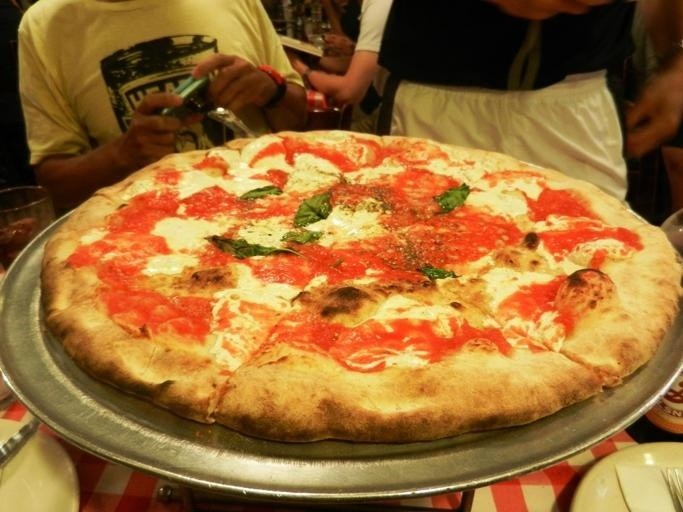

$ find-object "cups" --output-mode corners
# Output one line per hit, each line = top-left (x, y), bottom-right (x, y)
(2, 186), (54, 261)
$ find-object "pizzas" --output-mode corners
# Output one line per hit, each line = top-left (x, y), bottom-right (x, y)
(40, 128), (682, 443)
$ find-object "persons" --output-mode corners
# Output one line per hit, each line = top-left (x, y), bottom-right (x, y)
(17, 1), (681, 221)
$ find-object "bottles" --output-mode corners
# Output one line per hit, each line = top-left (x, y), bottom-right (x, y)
(642, 201), (682, 432)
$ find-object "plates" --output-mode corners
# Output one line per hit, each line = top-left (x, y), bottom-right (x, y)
(571, 441), (681, 512)
(0, 418), (83, 512)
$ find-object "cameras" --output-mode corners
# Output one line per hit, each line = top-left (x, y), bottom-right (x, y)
(158, 72), (213, 122)
